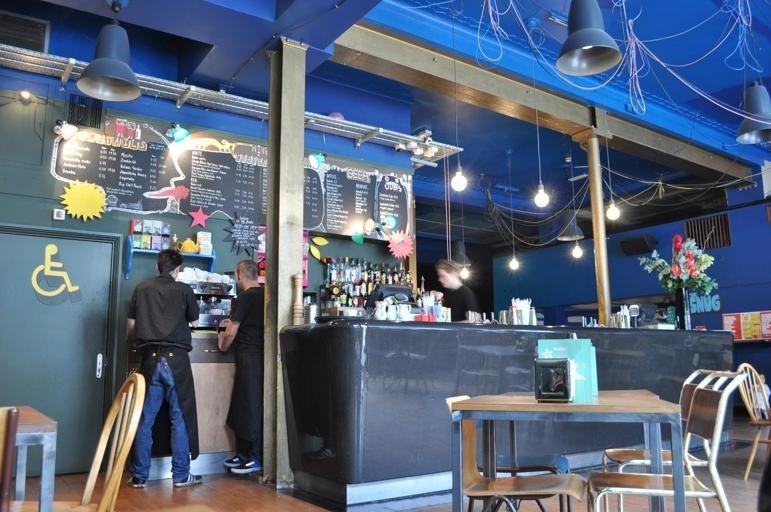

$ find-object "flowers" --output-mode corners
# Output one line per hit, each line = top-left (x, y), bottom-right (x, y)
(638, 233), (719, 296)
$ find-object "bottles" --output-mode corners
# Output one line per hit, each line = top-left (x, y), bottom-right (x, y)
(171, 234), (178, 253)
(509, 305), (519, 327)
(415, 288), (421, 307)
(619, 305), (630, 328)
(324, 256), (410, 307)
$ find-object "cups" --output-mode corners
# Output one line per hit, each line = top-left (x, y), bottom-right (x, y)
(373, 301), (388, 321)
(498, 310), (507, 325)
(397, 304), (411, 321)
(388, 304), (400, 320)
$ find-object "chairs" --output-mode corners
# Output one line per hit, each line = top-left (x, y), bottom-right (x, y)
(585, 369), (749, 511)
(0, 407), (19, 512)
(9, 373), (146, 512)
(445, 394), (590, 511)
(736, 363), (770, 483)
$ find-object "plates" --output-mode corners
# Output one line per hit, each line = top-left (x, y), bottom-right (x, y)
(313, 315), (364, 324)
(304, 312), (317, 325)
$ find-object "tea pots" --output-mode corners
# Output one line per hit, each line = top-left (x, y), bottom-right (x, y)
(178, 238), (199, 254)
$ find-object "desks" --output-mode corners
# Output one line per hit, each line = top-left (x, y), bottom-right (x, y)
(452, 387), (687, 511)
(0, 405), (58, 512)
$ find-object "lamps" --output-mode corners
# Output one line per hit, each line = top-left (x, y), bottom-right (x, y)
(170, 122), (189, 142)
(352, 216), (396, 244)
(555, 1), (622, 77)
(76, 0), (142, 103)
(556, 209), (584, 242)
(309, 133), (328, 169)
(57, 120), (78, 140)
(736, 57), (771, 145)
(453, 241), (471, 268)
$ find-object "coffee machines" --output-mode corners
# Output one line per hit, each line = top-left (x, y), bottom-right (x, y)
(187, 281), (236, 330)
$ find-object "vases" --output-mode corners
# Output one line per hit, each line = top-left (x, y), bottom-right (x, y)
(677, 288), (691, 333)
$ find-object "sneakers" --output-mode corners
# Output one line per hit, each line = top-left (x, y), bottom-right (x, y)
(224, 456), (243, 467)
(231, 460), (263, 474)
(127, 477), (146, 488)
(173, 473), (203, 486)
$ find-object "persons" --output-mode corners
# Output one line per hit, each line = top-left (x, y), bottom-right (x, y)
(754, 374), (771, 421)
(127, 249), (206, 488)
(435, 258), (477, 322)
(217, 259), (264, 476)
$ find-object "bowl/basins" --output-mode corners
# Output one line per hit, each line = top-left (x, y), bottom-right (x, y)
(175, 267), (231, 282)
